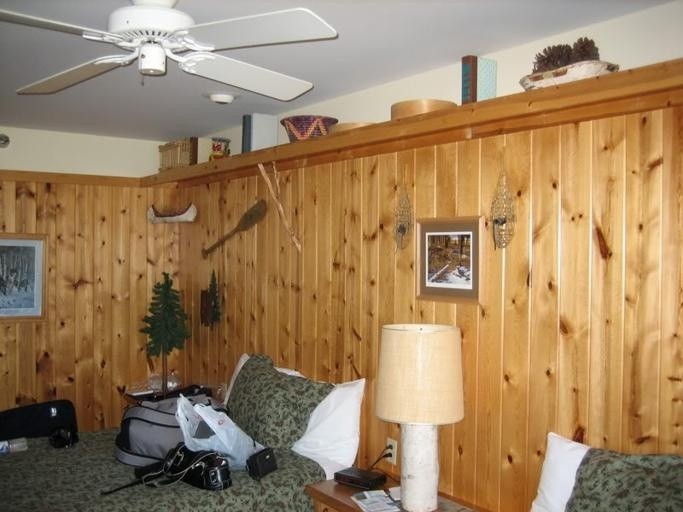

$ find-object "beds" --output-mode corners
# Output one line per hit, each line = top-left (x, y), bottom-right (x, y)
(0, 425), (340, 511)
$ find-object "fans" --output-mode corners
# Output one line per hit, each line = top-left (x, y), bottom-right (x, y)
(0, 0), (338, 102)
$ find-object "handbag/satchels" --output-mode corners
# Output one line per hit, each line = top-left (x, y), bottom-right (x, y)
(165, 440), (232, 490)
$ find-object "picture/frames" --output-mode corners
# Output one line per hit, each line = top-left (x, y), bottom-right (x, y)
(415, 217), (480, 304)
(0, 231), (48, 322)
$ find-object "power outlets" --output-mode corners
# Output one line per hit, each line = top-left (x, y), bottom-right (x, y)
(384, 437), (397, 467)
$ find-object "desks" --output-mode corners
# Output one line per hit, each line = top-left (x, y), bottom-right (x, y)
(124, 387), (211, 408)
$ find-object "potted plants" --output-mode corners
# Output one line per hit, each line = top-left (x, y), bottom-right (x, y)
(520, 36), (621, 91)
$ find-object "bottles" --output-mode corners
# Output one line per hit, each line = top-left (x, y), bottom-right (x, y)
(0, 437), (28, 454)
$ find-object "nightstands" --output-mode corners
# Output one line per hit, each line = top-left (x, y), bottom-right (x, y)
(304, 479), (490, 512)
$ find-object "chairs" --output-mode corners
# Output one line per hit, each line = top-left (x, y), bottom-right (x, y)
(1, 399), (77, 440)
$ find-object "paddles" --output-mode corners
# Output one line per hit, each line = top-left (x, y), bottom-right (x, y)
(201, 201), (266, 259)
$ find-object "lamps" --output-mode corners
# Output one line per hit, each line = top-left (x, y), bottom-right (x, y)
(375, 323), (466, 512)
(492, 171), (517, 249)
(392, 178), (412, 250)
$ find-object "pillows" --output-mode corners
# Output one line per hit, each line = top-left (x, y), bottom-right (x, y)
(222, 351), (366, 481)
(523, 432), (683, 512)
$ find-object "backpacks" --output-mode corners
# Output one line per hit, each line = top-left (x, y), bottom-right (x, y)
(115, 393), (229, 467)
